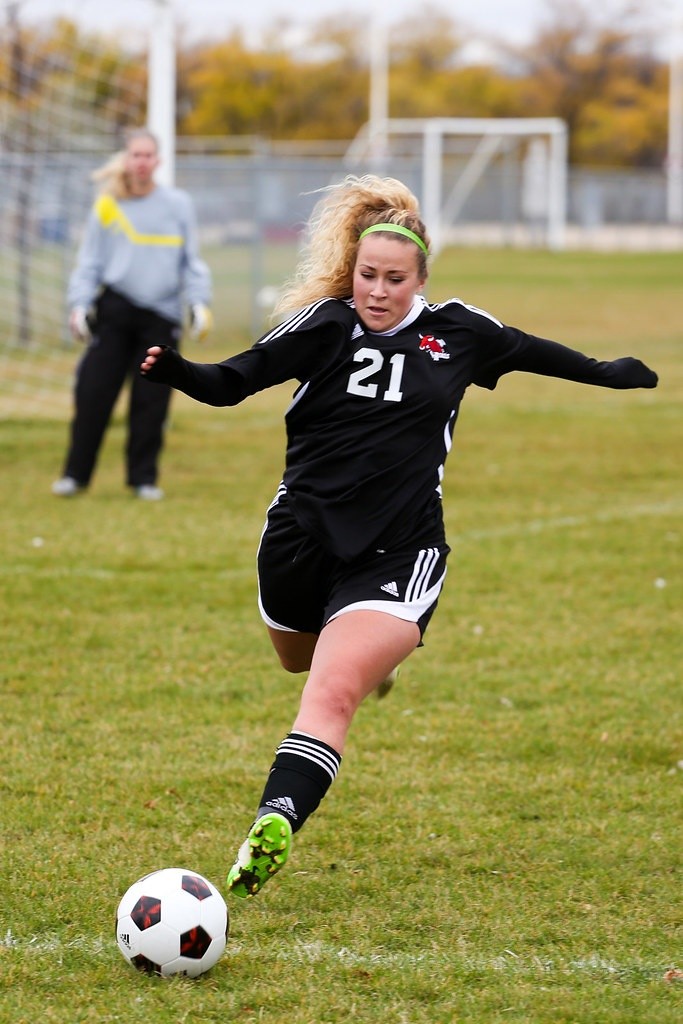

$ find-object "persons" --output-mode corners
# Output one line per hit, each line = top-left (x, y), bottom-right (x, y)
(140, 172), (659, 898)
(51, 130), (211, 499)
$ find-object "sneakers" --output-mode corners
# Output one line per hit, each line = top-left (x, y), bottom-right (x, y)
(135, 485), (163, 500)
(50, 476), (81, 496)
(226, 810), (292, 898)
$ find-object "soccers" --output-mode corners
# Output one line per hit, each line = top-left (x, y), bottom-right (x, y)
(115, 867), (231, 978)
